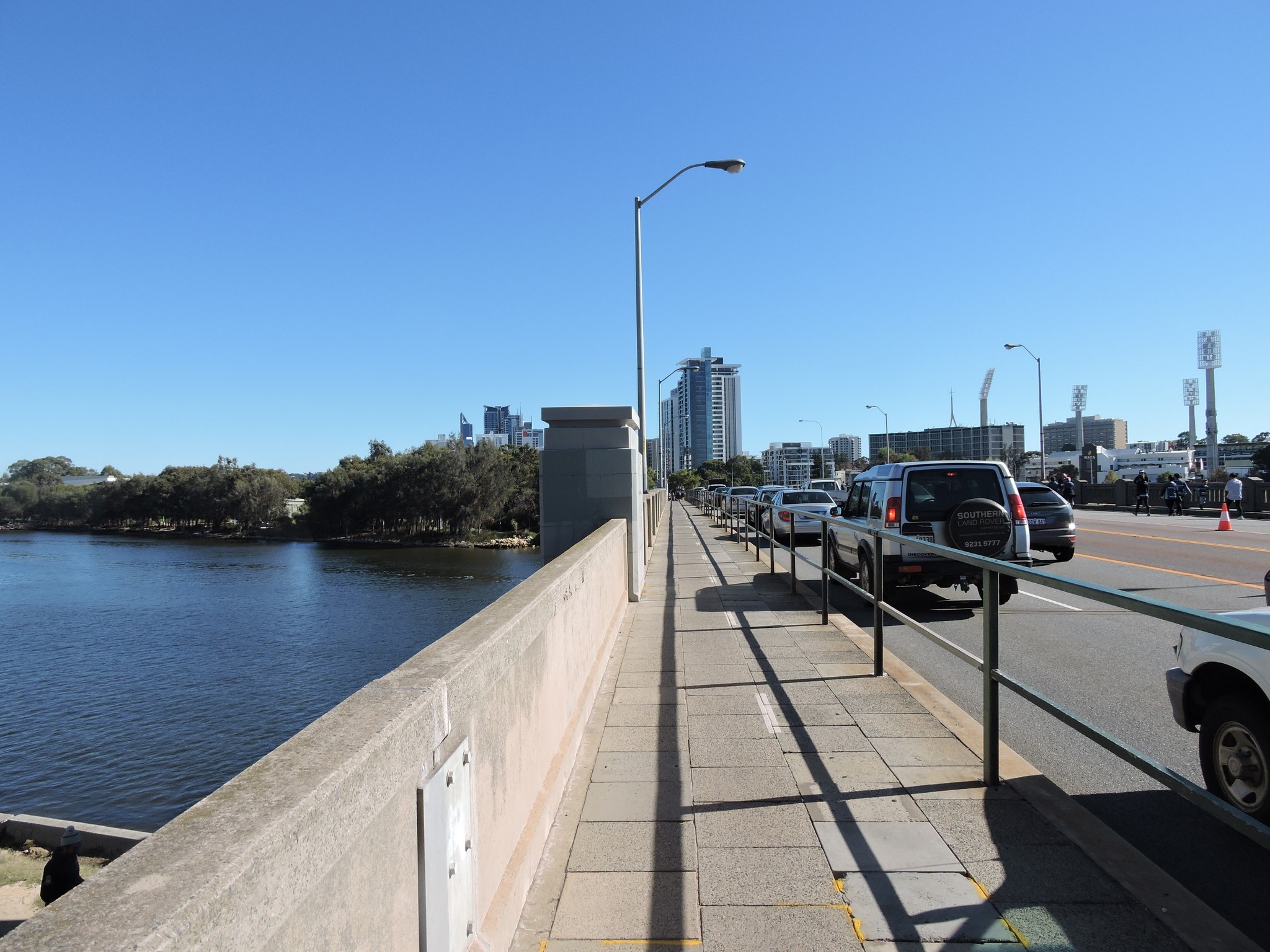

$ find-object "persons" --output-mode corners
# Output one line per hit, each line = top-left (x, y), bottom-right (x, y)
(1224, 473), (1244, 519)
(668, 486), (686, 502)
(1047, 473), (1074, 509)
(841, 481), (847, 490)
(1134, 470), (1209, 517)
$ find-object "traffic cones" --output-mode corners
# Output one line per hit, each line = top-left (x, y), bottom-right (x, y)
(1214, 503), (1234, 531)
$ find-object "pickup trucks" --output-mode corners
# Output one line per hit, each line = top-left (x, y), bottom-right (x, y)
(799, 478), (849, 506)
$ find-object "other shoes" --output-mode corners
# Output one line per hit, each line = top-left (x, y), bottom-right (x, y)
(1168, 513), (1183, 516)
(1132, 512), (1137, 516)
(1147, 514), (1150, 516)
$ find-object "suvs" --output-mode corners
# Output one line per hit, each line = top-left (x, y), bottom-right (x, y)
(828, 461), (1033, 613)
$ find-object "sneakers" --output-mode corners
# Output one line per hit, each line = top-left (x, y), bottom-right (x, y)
(1237, 516), (1244, 520)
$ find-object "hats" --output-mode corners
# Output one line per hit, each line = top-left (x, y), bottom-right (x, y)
(1139, 470), (1144, 473)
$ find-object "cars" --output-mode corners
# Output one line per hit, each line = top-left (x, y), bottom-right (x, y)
(692, 484), (837, 545)
(1166, 571), (1270, 819)
(993, 482), (1076, 562)
(909, 482), (935, 503)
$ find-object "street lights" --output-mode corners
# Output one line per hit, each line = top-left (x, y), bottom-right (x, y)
(866, 405), (890, 464)
(732, 461), (744, 488)
(1004, 344), (1045, 481)
(799, 420), (824, 489)
(635, 161), (745, 493)
(783, 443), (810, 489)
(656, 366), (700, 488)
(978, 368), (995, 425)
(1196, 329), (1223, 479)
(1070, 384), (1088, 451)
(1182, 378), (1200, 450)
